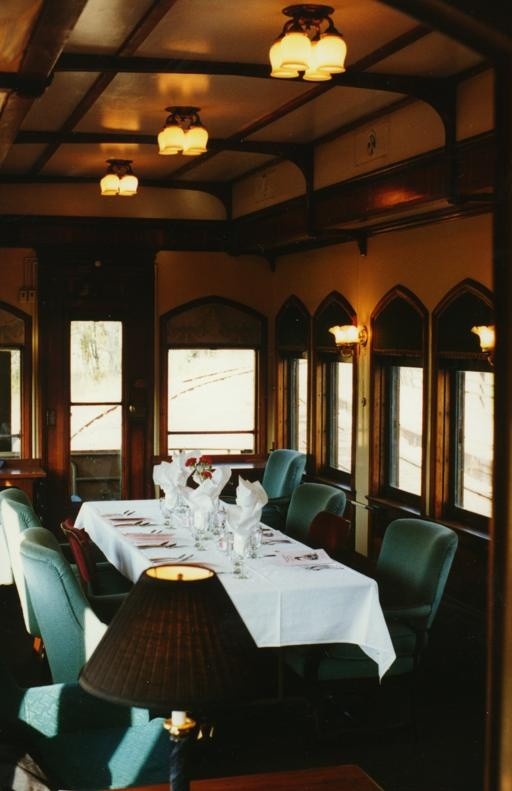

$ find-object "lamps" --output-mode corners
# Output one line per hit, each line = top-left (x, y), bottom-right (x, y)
(265, 2), (354, 86)
(327, 321), (370, 358)
(98, 156), (140, 196)
(78, 563), (269, 790)
(156, 102), (213, 159)
(471, 321), (495, 367)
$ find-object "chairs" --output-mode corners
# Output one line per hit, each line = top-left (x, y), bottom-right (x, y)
(322, 517), (459, 691)
(282, 482), (347, 538)
(0, 487), (42, 597)
(2, 683), (168, 790)
(261, 447), (311, 527)
(17, 526), (130, 684)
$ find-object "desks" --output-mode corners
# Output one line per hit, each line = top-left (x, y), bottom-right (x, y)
(80, 498), (377, 691)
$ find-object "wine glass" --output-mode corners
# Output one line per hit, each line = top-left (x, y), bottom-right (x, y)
(157, 490), (263, 577)
